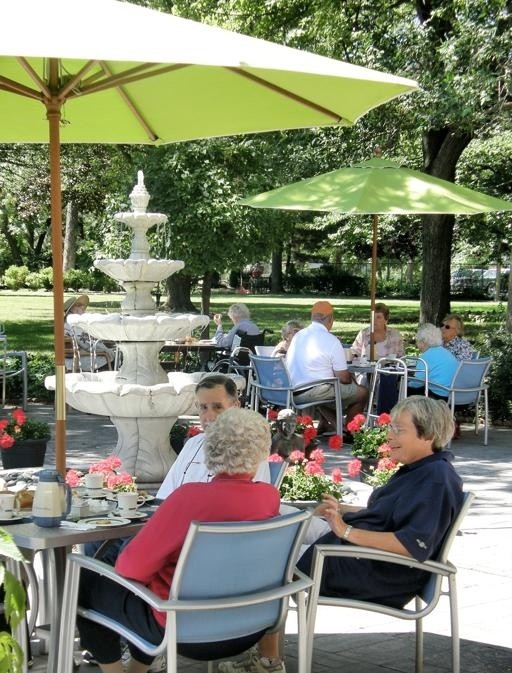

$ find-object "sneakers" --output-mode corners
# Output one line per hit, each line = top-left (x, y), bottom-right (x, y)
(122, 652), (166, 673)
(217, 651), (287, 673)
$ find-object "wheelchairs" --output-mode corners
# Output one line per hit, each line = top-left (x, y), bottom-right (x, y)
(210, 327), (275, 401)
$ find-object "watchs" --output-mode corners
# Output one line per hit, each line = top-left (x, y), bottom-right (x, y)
(342, 525), (353, 541)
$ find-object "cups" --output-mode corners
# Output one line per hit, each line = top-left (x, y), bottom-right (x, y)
(85, 473), (104, 489)
(1, 493), (16, 513)
(117, 491), (145, 517)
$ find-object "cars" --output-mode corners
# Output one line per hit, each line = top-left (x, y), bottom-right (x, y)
(457, 268), (511, 300)
(450, 268), (488, 292)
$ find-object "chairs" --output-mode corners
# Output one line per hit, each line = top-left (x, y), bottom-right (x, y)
(56, 507), (313, 672)
(266, 461), (290, 491)
(60, 336), (116, 371)
(0, 333), (28, 412)
(245, 354), (344, 448)
(399, 356), (493, 449)
(305, 490), (476, 672)
(467, 350), (481, 360)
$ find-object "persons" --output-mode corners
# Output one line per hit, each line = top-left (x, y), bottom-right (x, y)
(81, 375), (271, 558)
(286, 302), (368, 444)
(441, 315), (472, 363)
(349, 303), (404, 358)
(64, 295), (114, 372)
(213, 303), (260, 350)
(270, 321), (304, 358)
(407, 323), (458, 403)
(72, 408), (281, 672)
(221, 394), (463, 673)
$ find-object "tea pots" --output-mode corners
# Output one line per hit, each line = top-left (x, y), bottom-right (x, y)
(32, 469), (72, 528)
(366, 341), (378, 362)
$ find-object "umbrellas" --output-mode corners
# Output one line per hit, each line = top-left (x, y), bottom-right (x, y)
(234, 147), (512, 362)
(0, 1), (423, 470)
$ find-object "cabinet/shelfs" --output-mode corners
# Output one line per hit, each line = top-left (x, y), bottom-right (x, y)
(364, 356), (429, 432)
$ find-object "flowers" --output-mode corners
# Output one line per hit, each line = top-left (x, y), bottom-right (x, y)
(63, 454), (137, 494)
(347, 445), (405, 487)
(328, 412), (396, 457)
(1, 409), (56, 448)
(267, 449), (344, 502)
(168, 420), (203, 454)
(252, 408), (318, 450)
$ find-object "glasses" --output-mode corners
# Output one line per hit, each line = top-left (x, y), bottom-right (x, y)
(386, 423), (406, 434)
(440, 323), (451, 329)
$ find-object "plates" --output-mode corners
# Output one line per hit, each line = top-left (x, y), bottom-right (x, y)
(0, 515), (23, 522)
(107, 494), (155, 502)
(77, 517), (130, 527)
(108, 511), (148, 519)
(200, 340), (214, 343)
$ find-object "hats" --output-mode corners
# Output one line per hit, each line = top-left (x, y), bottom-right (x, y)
(311, 300), (333, 319)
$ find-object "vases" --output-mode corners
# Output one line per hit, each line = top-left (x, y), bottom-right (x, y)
(357, 458), (381, 483)
(1, 435), (53, 470)
